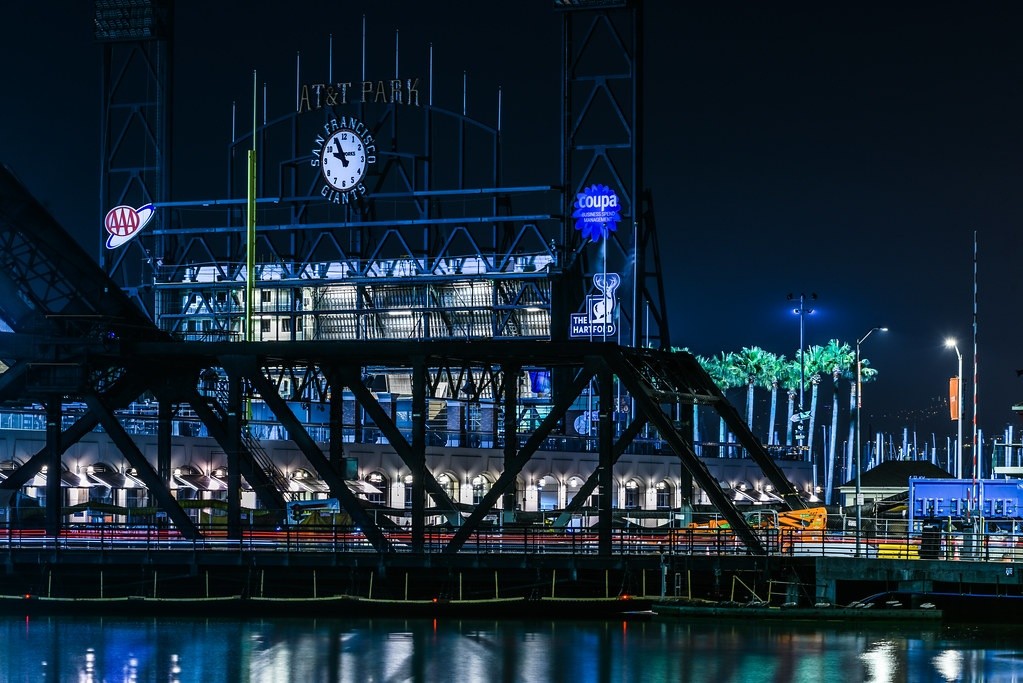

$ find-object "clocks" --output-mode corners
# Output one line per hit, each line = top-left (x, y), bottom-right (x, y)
(306, 113), (378, 208)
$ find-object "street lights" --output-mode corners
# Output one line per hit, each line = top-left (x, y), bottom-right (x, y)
(788, 293), (817, 464)
(941, 337), (967, 478)
(854, 323), (888, 557)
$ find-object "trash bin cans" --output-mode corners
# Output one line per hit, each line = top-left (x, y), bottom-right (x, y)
(919, 517), (943, 559)
